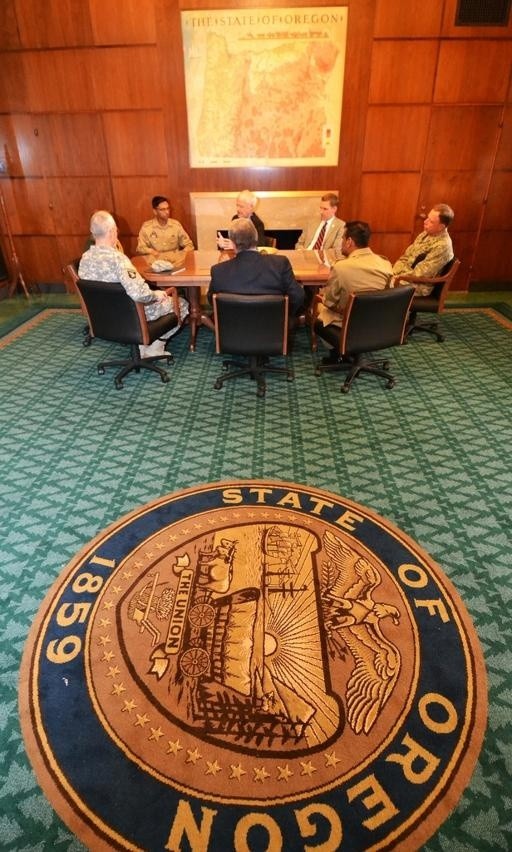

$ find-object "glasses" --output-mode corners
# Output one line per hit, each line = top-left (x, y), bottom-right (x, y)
(153, 206), (171, 211)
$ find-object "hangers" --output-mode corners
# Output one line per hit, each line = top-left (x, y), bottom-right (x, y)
(0, 186), (34, 305)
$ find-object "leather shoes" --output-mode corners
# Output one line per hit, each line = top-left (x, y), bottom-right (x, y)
(344, 354), (359, 366)
(310, 353), (345, 369)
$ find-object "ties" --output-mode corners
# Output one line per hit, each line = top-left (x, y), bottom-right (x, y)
(312, 221), (328, 250)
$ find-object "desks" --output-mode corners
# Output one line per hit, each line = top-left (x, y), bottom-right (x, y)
(123, 247), (393, 352)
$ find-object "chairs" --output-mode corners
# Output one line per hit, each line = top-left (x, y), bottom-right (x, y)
(66, 261), (94, 348)
(73, 278), (181, 386)
(210, 292), (294, 397)
(391, 256), (462, 344)
(265, 235), (278, 250)
(315, 285), (417, 393)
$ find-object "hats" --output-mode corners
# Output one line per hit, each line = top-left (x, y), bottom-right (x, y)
(143, 259), (174, 274)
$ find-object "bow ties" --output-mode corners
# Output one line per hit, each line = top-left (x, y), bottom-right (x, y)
(318, 250), (327, 266)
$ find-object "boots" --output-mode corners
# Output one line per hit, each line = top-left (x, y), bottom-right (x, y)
(145, 339), (173, 361)
(138, 344), (147, 360)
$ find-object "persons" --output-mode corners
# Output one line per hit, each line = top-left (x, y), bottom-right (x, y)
(207, 219), (305, 320)
(315, 221), (394, 328)
(217, 190), (265, 253)
(135, 196), (194, 259)
(394, 204), (453, 297)
(296, 193), (348, 249)
(78, 210), (189, 361)
